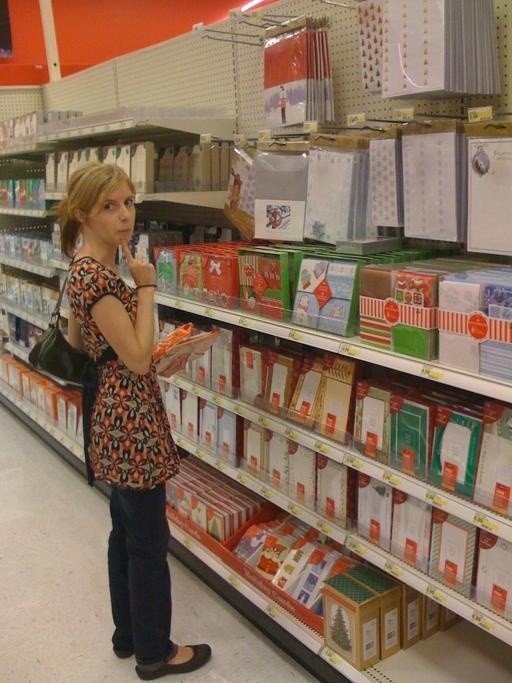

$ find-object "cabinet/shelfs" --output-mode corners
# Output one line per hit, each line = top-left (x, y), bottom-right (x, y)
(35, 118), (237, 498)
(1, 144), (53, 434)
(92, 257), (511, 683)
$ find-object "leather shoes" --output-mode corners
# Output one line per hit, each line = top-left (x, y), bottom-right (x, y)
(136, 642), (212, 681)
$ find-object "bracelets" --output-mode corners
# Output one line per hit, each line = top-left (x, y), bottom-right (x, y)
(135, 284), (160, 293)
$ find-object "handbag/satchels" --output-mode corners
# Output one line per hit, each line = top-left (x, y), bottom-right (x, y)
(29, 263), (109, 399)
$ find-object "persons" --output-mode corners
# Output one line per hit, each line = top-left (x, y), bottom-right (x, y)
(48, 163), (212, 680)
